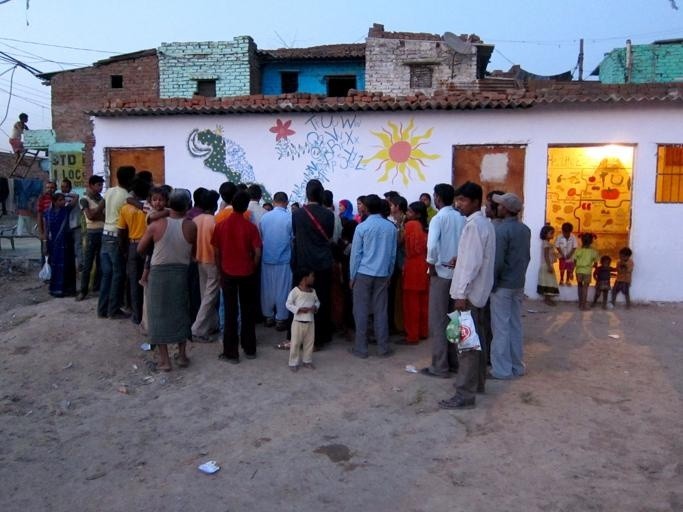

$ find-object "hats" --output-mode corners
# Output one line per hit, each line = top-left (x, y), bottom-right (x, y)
(491, 192), (522, 214)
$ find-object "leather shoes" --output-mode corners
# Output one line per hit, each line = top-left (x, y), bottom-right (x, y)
(337, 328), (428, 359)
(437, 396), (476, 410)
(420, 368), (448, 378)
(76, 289), (89, 301)
(453, 383), (486, 394)
(107, 311), (132, 319)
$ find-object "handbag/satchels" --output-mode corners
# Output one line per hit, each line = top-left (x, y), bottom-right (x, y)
(329, 262), (344, 326)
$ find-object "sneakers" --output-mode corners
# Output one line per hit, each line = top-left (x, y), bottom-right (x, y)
(219, 354), (240, 364)
(245, 352), (257, 359)
(192, 334), (213, 343)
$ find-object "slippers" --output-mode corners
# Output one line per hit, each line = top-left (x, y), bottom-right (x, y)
(146, 361), (169, 373)
(275, 338), (291, 349)
(169, 352), (187, 369)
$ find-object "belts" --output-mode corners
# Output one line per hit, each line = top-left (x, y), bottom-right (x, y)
(129, 239), (140, 243)
(103, 231), (118, 237)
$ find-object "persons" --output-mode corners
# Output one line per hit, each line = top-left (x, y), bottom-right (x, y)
(591, 255), (613, 310)
(609, 247), (634, 309)
(573, 231), (600, 311)
(555, 221), (578, 286)
(34, 163), (533, 410)
(8, 112), (30, 165)
(536, 225), (561, 306)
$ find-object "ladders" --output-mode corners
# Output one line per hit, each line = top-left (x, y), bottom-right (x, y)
(8, 150), (39, 180)
